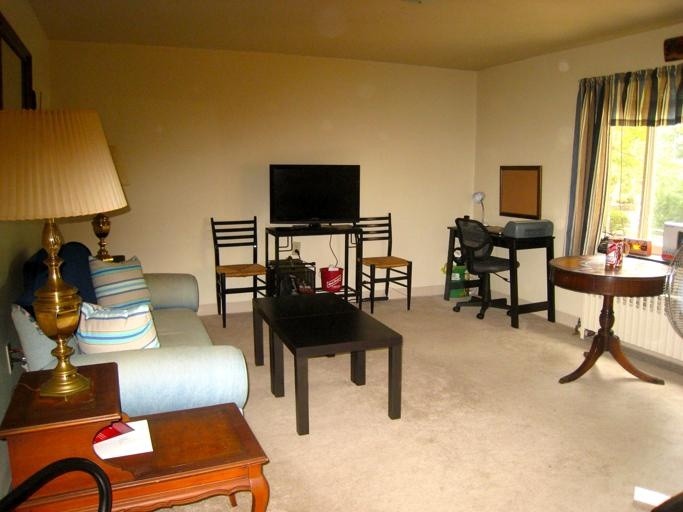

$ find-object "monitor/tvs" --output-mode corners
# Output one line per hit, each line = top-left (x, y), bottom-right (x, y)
(268, 164), (360, 231)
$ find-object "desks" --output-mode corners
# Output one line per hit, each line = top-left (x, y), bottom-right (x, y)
(443, 226), (555, 328)
(547, 252), (675, 385)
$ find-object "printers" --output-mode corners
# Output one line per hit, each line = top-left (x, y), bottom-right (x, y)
(503, 219), (553, 238)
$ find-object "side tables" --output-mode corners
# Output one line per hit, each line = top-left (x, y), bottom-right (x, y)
(6, 402), (272, 512)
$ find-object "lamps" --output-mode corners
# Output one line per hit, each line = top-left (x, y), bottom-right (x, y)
(471, 191), (488, 227)
(0, 106), (128, 399)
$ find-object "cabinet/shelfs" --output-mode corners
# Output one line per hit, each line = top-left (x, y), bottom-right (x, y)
(264, 225), (363, 311)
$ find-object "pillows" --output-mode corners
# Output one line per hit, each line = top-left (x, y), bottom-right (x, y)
(76, 257), (160, 355)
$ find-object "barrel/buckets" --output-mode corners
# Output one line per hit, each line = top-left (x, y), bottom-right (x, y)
(447, 266), (471, 298)
(320, 266), (343, 291)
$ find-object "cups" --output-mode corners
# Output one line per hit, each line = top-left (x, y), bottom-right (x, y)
(605, 238), (630, 269)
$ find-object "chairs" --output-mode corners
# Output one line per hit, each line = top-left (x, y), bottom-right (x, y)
(452, 218), (519, 320)
(352, 213), (412, 314)
(210, 216), (270, 328)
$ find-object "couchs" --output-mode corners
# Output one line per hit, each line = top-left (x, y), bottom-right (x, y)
(11, 241), (247, 419)
